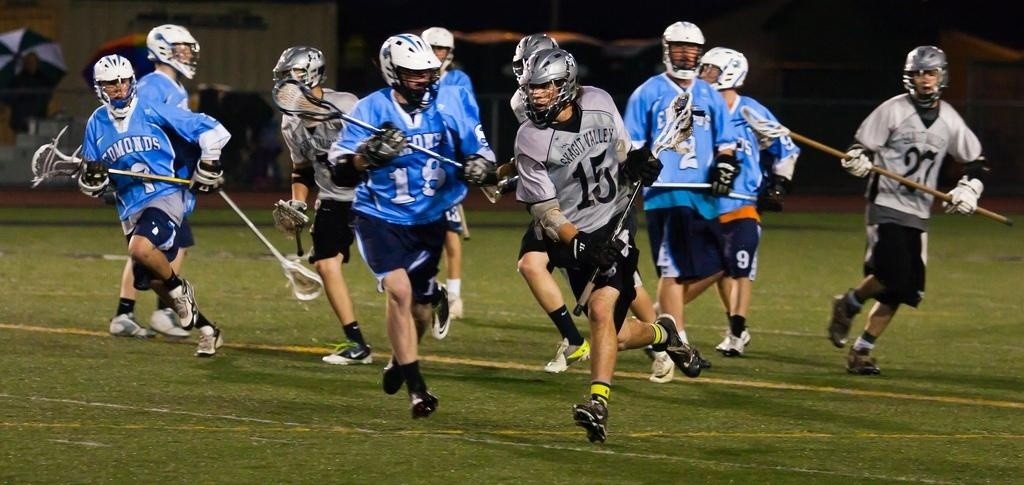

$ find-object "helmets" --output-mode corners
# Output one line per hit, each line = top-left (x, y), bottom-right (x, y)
(91, 54), (137, 111)
(902, 46), (948, 101)
(273, 45), (327, 90)
(519, 48), (578, 124)
(511, 34), (560, 81)
(146, 24), (200, 79)
(378, 33), (441, 107)
(661, 21), (706, 81)
(421, 26), (455, 51)
(699, 47), (750, 92)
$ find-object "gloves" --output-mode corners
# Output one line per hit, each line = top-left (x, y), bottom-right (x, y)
(357, 126), (408, 170)
(570, 230), (619, 269)
(939, 176), (985, 216)
(274, 200), (309, 232)
(627, 148), (664, 187)
(460, 154), (497, 185)
(841, 148), (873, 180)
(83, 161), (110, 186)
(188, 160), (221, 195)
(757, 173), (790, 212)
(712, 156), (739, 197)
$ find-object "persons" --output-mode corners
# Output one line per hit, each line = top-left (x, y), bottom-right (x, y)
(514, 49), (703, 443)
(695, 47), (802, 358)
(326, 33), (498, 417)
(272, 47), (453, 364)
(620, 18), (737, 369)
(4, 52), (52, 146)
(110, 25), (201, 338)
(830, 46), (986, 374)
(495, 34), (675, 382)
(190, 85), (285, 193)
(76, 55), (234, 359)
(420, 27), (481, 321)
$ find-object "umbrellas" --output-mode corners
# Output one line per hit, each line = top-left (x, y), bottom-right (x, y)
(0, 29), (65, 103)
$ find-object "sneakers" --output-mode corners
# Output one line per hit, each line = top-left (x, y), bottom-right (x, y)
(544, 337), (591, 375)
(194, 328), (226, 356)
(108, 310), (157, 338)
(321, 341), (374, 367)
(409, 391), (439, 419)
(149, 309), (191, 338)
(171, 279), (199, 331)
(654, 313), (702, 377)
(846, 343), (882, 377)
(716, 327), (752, 355)
(649, 350), (675, 383)
(382, 359), (404, 394)
(449, 295), (463, 320)
(571, 399), (609, 443)
(829, 289), (860, 349)
(428, 283), (450, 339)
